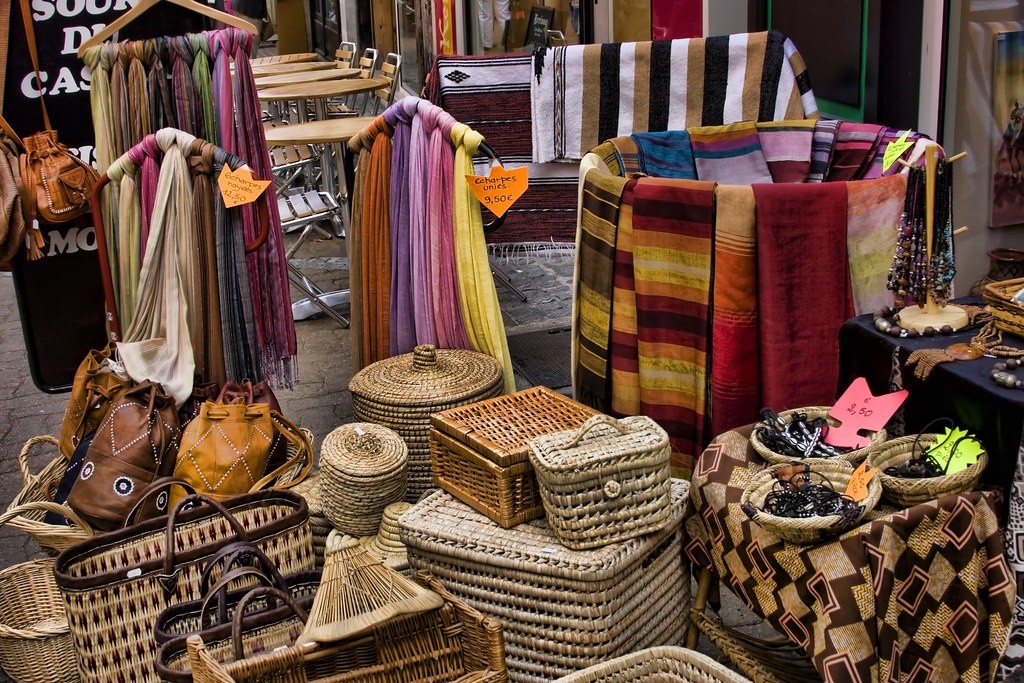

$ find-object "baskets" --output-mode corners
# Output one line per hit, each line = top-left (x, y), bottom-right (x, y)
(398, 386), (748, 682)
(188, 571), (510, 682)
(4, 428), (312, 551)
(1, 501), (99, 682)
(741, 405), (989, 543)
(984, 279), (1024, 337)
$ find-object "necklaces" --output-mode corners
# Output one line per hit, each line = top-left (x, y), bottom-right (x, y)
(873, 304), (1024, 390)
(752, 406), (984, 519)
(886, 157), (957, 309)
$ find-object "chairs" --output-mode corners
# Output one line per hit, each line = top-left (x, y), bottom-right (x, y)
(315, 53), (404, 185)
(228, 102), (342, 259)
(293, 48), (378, 124)
(287, 42), (357, 108)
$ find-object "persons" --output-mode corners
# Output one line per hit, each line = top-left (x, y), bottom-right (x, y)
(478, 0), (510, 56)
(230, 0), (269, 59)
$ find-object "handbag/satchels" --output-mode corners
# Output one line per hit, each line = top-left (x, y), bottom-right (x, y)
(153, 542), (323, 683)
(52, 477), (316, 683)
(62, 341), (313, 530)
(1, 116), (101, 272)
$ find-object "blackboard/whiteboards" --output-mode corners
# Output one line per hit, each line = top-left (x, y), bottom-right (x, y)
(527, 5), (555, 45)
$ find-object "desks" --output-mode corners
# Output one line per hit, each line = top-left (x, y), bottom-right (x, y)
(228, 52), (318, 117)
(263, 116), (379, 265)
(835, 293), (1024, 526)
(255, 67), (361, 191)
(251, 60), (336, 125)
(257, 79), (389, 238)
